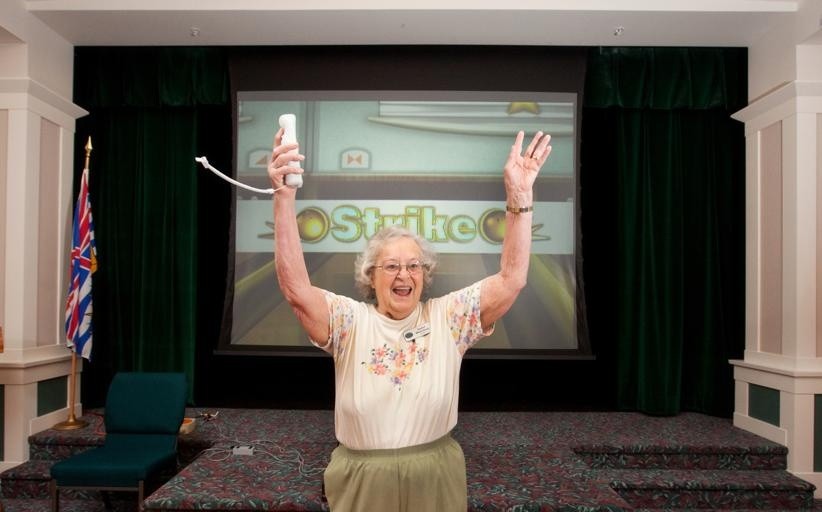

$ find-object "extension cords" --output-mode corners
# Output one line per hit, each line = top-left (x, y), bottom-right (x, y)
(179, 417), (197, 434)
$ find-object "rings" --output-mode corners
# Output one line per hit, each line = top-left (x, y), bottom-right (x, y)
(532, 156), (539, 160)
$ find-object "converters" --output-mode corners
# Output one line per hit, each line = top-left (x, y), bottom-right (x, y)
(233, 445), (256, 456)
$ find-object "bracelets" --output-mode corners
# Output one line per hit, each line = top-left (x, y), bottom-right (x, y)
(505, 206), (533, 213)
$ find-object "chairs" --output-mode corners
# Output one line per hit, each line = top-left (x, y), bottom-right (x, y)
(48, 370), (192, 512)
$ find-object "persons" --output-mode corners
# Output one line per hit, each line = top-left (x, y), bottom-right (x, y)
(264, 125), (552, 512)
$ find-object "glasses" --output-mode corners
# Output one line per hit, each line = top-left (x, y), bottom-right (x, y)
(370, 258), (426, 277)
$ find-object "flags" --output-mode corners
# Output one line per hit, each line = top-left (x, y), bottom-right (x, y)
(64, 168), (97, 362)
